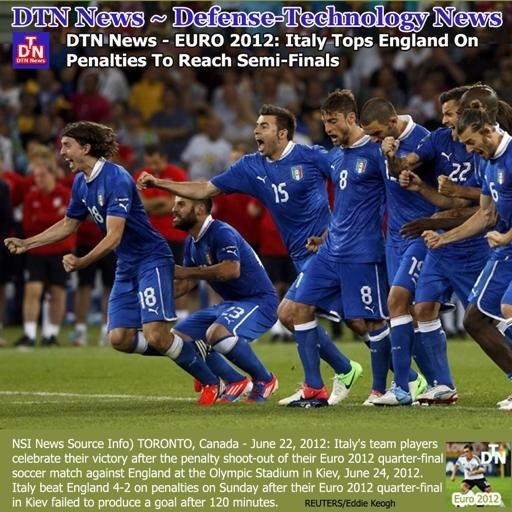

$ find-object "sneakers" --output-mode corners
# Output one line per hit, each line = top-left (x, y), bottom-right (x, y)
(0, 331), (110, 349)
(190, 339), (211, 364)
(438, 302), (457, 315)
(446, 330), (470, 340)
(327, 360), (363, 406)
(194, 373), (278, 406)
(363, 372), (458, 407)
(271, 334), (295, 342)
(496, 396), (512, 410)
(279, 383), (330, 407)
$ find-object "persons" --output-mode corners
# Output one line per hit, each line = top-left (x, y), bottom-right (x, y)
(450, 443), (493, 496)
(0, 0), (512, 410)
